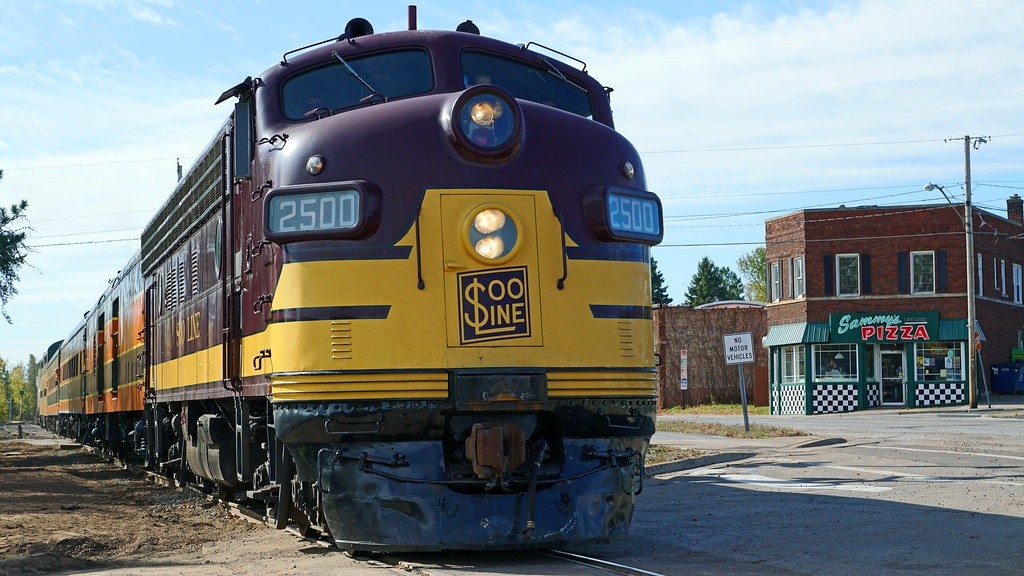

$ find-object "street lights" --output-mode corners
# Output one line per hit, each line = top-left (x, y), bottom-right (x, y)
(924, 181), (978, 410)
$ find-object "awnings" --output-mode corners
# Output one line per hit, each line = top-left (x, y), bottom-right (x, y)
(761, 321), (830, 345)
(939, 319), (986, 340)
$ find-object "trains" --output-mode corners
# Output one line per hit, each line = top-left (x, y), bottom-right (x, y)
(33, 3), (666, 559)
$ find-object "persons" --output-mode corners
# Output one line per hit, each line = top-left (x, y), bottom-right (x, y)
(304, 95), (327, 119)
(466, 68), (494, 87)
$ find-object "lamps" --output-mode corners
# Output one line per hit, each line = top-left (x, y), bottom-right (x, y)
(835, 345), (844, 359)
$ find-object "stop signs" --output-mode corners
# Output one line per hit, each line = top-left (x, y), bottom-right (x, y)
(972, 330), (982, 351)
